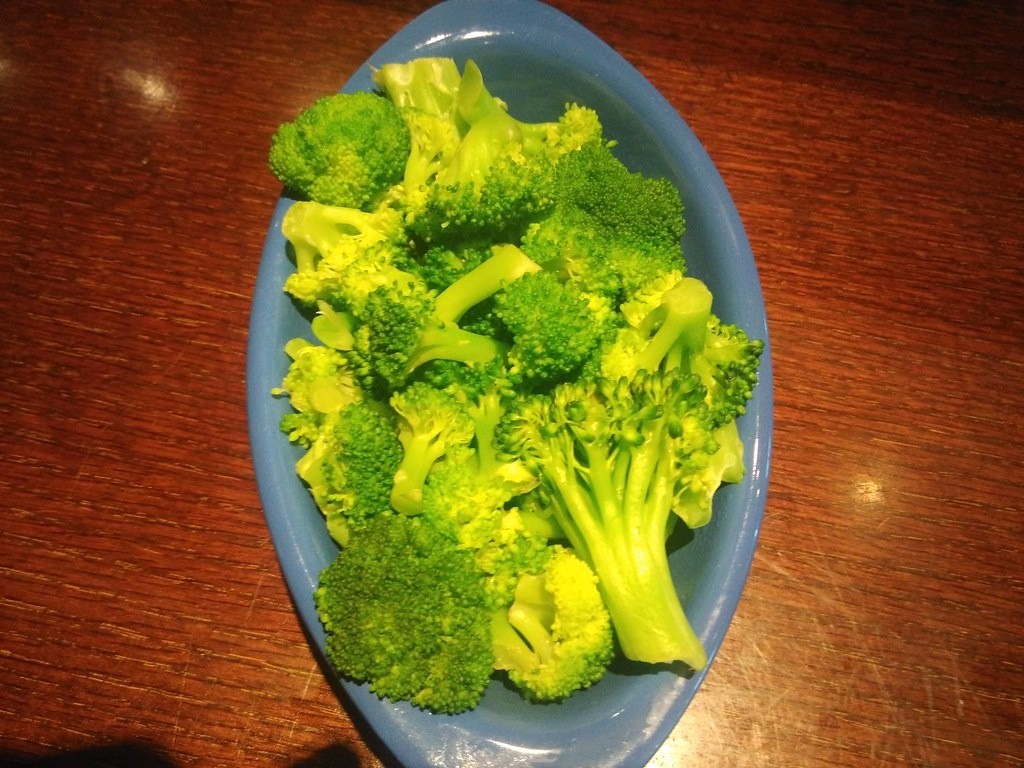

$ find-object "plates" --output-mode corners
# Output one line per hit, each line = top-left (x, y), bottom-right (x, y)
(240, 0), (776, 768)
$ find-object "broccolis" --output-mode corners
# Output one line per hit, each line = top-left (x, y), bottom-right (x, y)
(268, 57), (765, 716)
